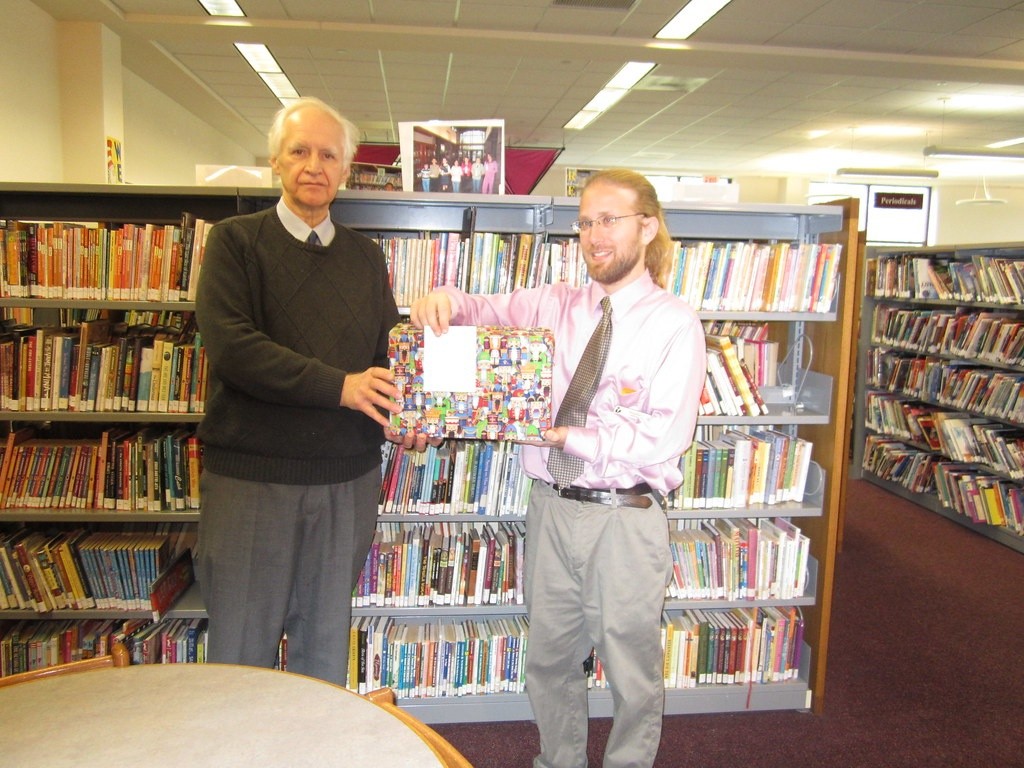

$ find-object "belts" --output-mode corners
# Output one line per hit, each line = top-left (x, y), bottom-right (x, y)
(546, 482), (653, 509)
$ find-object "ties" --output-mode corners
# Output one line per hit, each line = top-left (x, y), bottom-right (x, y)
(308, 230), (318, 245)
(547, 296), (614, 490)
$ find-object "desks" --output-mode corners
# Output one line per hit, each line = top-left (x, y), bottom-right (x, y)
(0, 665), (452, 768)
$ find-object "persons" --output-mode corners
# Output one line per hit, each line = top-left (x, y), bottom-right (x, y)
(411, 170), (705, 768)
(417, 151), (498, 194)
(197, 96), (441, 692)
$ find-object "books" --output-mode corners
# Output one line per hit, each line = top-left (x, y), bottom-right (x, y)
(861, 251), (1024, 539)
(2, 218), (843, 708)
(346, 165), (403, 191)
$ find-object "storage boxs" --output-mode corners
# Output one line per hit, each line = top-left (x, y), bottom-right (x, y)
(386, 322), (556, 443)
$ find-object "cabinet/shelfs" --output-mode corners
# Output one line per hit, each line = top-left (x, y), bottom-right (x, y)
(862, 241), (1024, 555)
(0, 182), (860, 725)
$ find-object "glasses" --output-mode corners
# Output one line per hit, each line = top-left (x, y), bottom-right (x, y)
(570, 212), (648, 233)
